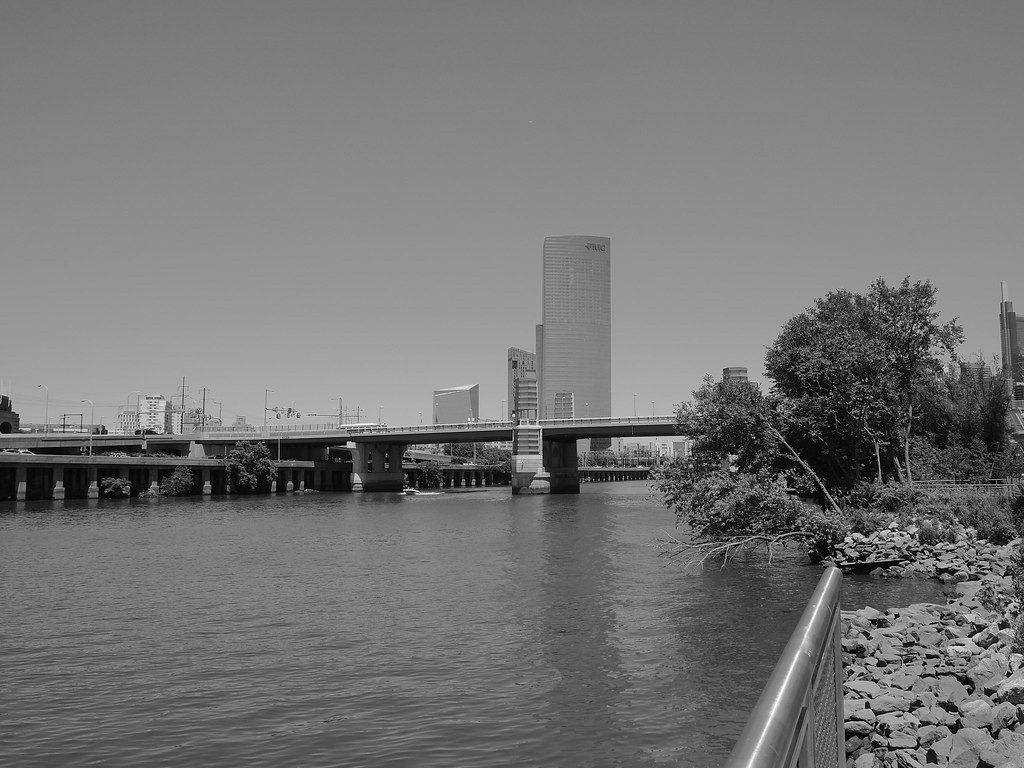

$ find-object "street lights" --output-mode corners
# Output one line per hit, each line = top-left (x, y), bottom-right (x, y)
(470, 409), (473, 423)
(419, 412), (423, 425)
(652, 400), (655, 419)
(561, 397), (567, 420)
(435, 402), (439, 425)
(502, 399), (506, 421)
(58, 416), (69, 433)
(633, 394), (638, 418)
(80, 399), (93, 457)
(263, 388), (274, 426)
(36, 384), (48, 433)
(377, 405), (384, 427)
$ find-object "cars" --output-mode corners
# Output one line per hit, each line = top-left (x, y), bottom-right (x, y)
(463, 461), (478, 466)
(0, 447), (36, 456)
(134, 429), (158, 435)
(207, 454), (233, 459)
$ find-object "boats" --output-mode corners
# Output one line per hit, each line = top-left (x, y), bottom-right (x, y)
(403, 488), (419, 496)
